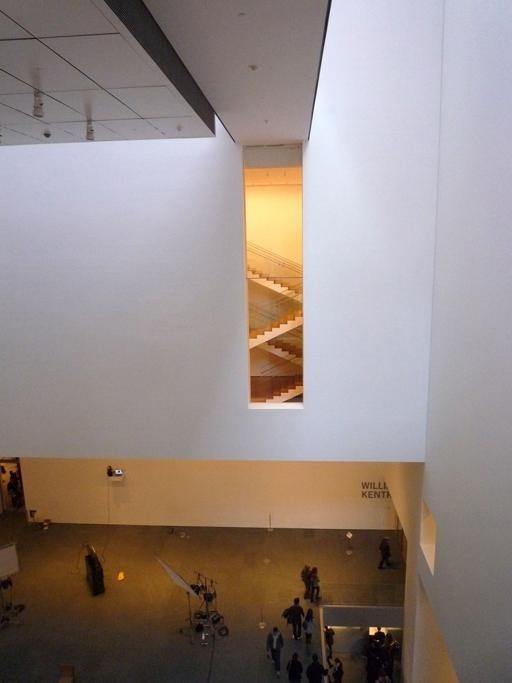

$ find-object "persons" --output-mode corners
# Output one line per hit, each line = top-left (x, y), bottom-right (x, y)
(265, 562), (393, 681)
(8, 469), (19, 505)
(377, 536), (393, 569)
